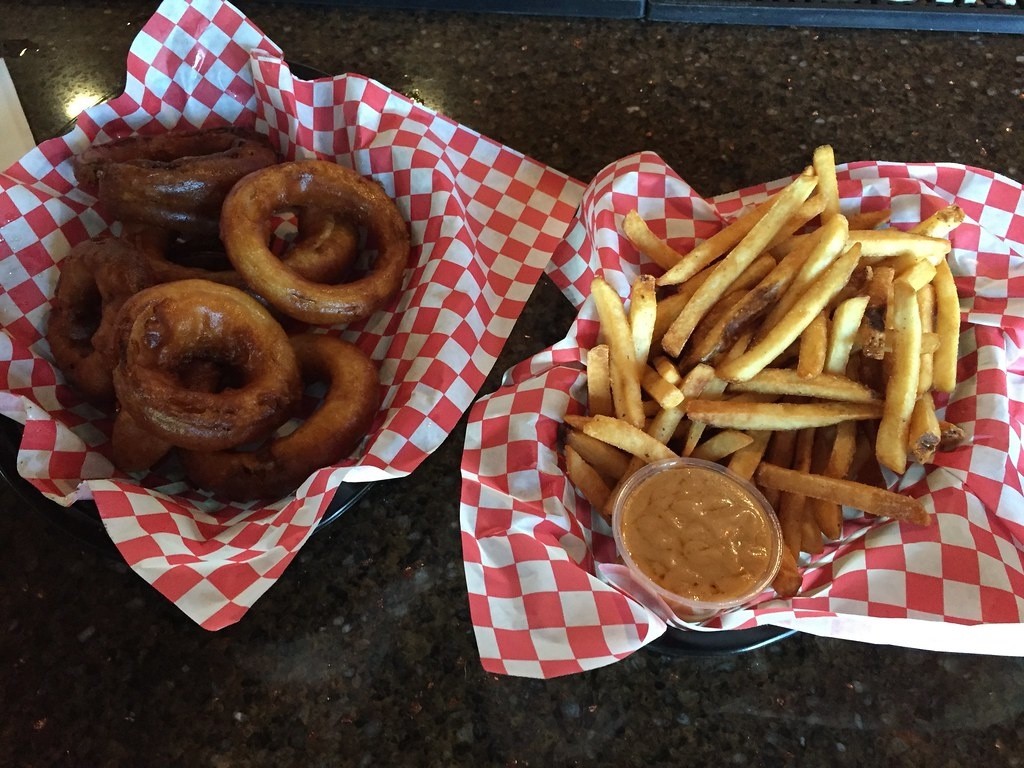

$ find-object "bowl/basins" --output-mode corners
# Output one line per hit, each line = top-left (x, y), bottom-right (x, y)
(612, 458), (783, 622)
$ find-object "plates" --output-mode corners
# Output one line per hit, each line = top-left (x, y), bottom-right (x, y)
(515, 301), (800, 653)
(0, 61), (374, 561)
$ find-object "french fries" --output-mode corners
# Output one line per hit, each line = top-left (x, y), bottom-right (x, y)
(562, 145), (971, 600)
